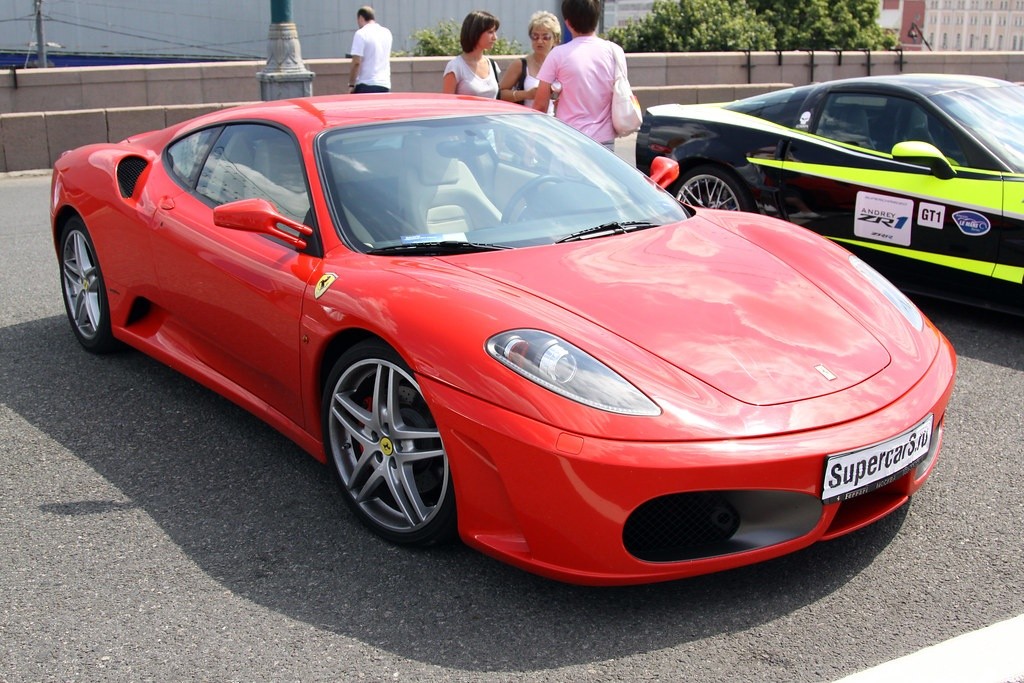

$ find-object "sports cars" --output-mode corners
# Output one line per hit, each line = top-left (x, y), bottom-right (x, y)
(49, 93), (959, 588)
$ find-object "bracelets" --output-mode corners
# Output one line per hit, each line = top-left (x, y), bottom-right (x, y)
(348, 84), (354, 87)
(513, 90), (518, 101)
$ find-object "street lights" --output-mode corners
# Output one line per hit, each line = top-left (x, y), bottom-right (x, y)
(908, 21), (932, 52)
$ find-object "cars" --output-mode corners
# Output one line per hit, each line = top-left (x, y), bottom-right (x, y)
(635, 72), (1024, 318)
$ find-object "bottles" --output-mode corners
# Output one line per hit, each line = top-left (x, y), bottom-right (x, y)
(550, 81), (562, 100)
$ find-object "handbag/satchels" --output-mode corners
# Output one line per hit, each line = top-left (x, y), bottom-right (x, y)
(611, 41), (643, 137)
(489, 59), (501, 100)
(513, 58), (527, 105)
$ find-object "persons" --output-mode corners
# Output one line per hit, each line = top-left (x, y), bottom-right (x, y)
(532, 0), (628, 152)
(499, 12), (560, 117)
(443, 10), (502, 155)
(347, 6), (393, 94)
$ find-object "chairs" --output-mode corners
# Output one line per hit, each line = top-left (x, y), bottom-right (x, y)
(830, 103), (876, 150)
(243, 137), (377, 250)
(400, 133), (502, 236)
(893, 104), (936, 147)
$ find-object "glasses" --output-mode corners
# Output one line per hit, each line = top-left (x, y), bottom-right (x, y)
(529, 32), (556, 40)
